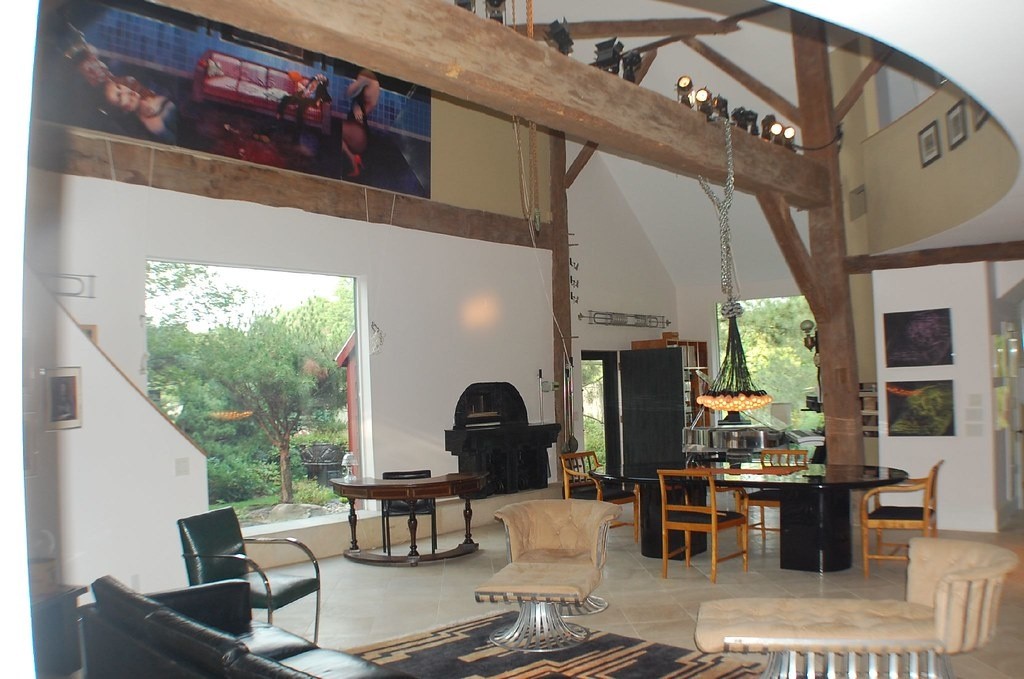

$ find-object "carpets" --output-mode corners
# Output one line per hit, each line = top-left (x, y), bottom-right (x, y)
(343, 610), (766, 679)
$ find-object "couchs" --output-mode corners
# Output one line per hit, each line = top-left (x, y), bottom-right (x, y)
(191, 50), (331, 134)
(76, 574), (416, 679)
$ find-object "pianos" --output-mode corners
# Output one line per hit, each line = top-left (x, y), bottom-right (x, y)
(681, 370), (792, 463)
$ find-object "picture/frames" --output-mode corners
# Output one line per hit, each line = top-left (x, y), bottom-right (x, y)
(43, 366), (82, 432)
(916, 120), (942, 169)
(944, 99), (969, 151)
(971, 96), (991, 132)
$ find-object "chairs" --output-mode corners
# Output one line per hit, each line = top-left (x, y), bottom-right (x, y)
(177, 506), (322, 645)
(473, 499), (623, 654)
(736, 449), (809, 549)
(656, 469), (749, 584)
(862, 459), (946, 581)
(381, 470), (437, 557)
(694, 537), (1020, 679)
(560, 451), (640, 546)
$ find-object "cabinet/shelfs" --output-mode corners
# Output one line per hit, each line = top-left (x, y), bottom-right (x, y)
(631, 339), (710, 427)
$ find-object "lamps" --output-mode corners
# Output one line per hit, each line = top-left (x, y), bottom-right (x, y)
(622, 50), (642, 83)
(695, 88), (714, 114)
(454, 0), (507, 25)
(731, 107), (748, 131)
(692, 295), (772, 413)
(713, 95), (730, 122)
(677, 76), (696, 108)
(800, 320), (817, 351)
(770, 122), (784, 146)
(783, 126), (796, 152)
(592, 36), (624, 76)
(761, 114), (776, 142)
(746, 110), (759, 137)
(543, 16), (575, 56)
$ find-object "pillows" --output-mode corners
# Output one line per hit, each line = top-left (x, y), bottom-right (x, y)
(207, 59), (226, 78)
(265, 87), (288, 102)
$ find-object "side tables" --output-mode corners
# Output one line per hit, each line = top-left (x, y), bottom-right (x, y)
(30, 576), (88, 679)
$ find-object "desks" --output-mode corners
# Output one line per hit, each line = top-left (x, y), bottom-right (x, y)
(329, 472), (490, 567)
(588, 458), (908, 573)
